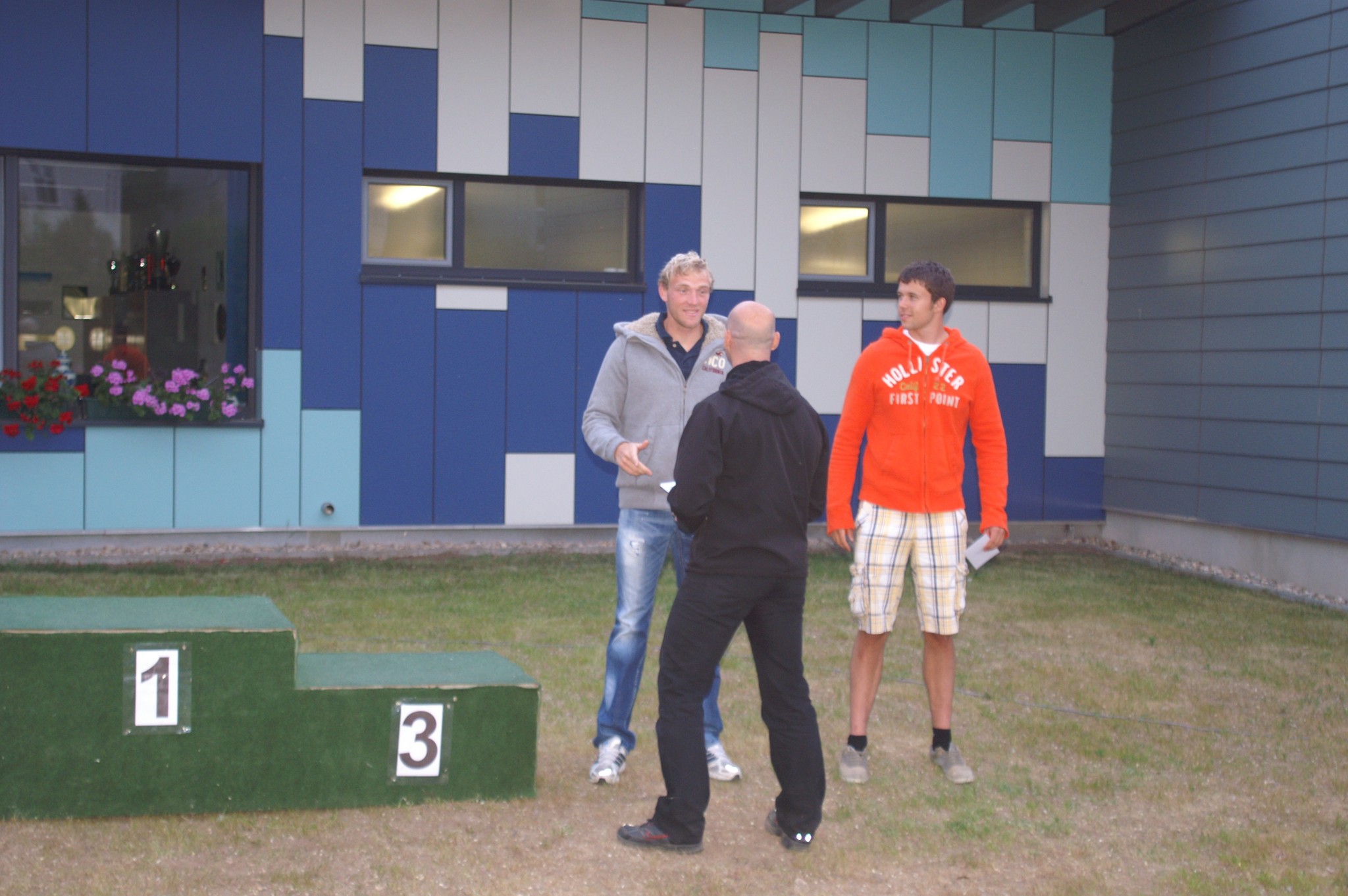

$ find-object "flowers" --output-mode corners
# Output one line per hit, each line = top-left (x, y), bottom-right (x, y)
(0, 355), (255, 438)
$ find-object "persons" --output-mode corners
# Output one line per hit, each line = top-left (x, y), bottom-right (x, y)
(580, 250), (742, 785)
(101, 317), (153, 383)
(826, 261), (1008, 784)
(617, 301), (830, 854)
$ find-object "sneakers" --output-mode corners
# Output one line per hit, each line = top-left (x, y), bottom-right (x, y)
(616, 819), (705, 854)
(764, 811), (814, 851)
(589, 735), (630, 786)
(705, 743), (743, 782)
(929, 743), (974, 784)
(839, 744), (871, 785)
